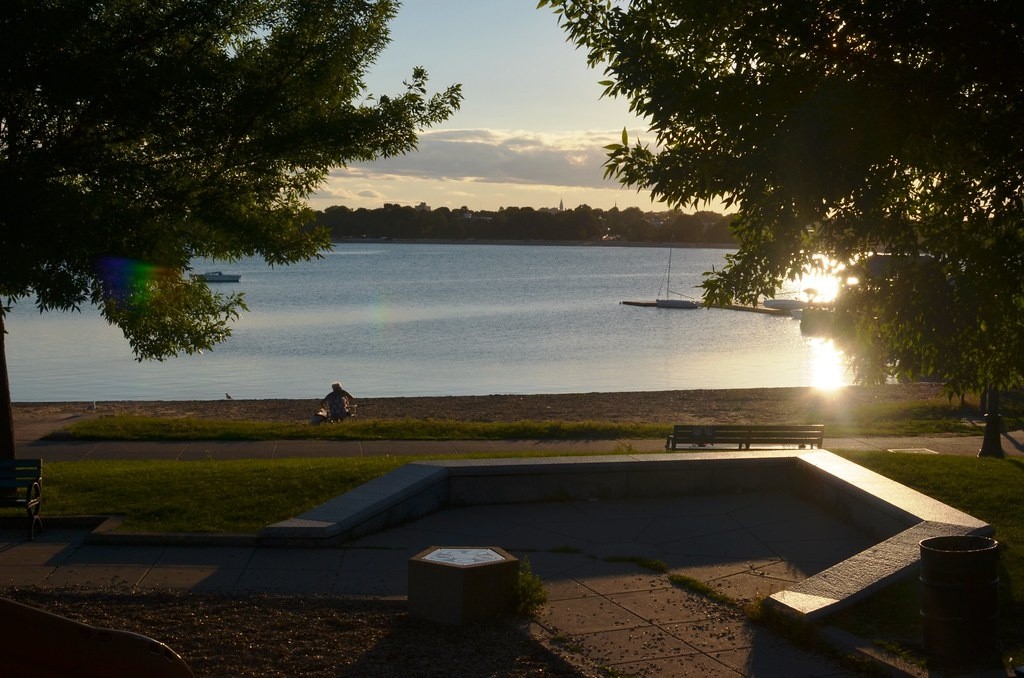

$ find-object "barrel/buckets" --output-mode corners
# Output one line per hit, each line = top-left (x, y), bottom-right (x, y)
(918, 535), (999, 674)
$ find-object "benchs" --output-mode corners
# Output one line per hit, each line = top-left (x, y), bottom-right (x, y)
(664, 424), (824, 453)
(0, 458), (43, 540)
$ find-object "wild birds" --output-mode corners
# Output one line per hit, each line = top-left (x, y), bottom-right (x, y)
(86, 401), (97, 413)
(226, 393), (233, 400)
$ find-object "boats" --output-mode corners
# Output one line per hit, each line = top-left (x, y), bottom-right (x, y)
(189, 271), (242, 281)
(763, 300), (804, 309)
(622, 234), (701, 309)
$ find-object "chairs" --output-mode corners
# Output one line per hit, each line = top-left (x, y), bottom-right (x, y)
(325, 396), (358, 423)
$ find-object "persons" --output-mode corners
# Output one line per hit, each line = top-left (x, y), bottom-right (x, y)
(320, 383), (358, 419)
